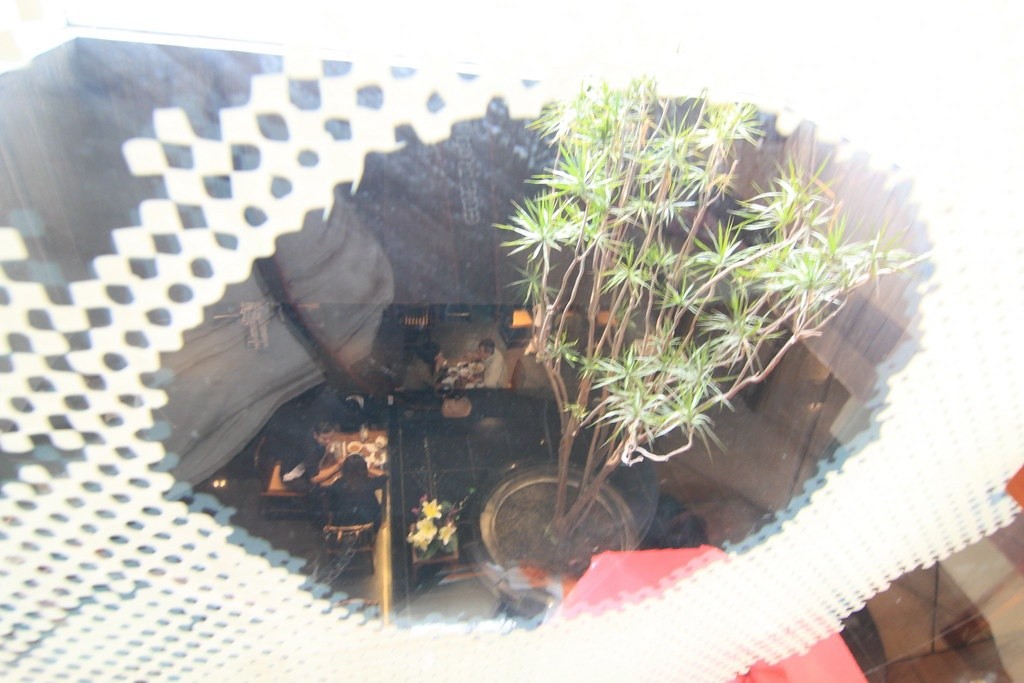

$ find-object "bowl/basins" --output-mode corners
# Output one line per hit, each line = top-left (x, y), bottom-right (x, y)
(442, 361), (485, 388)
(347, 440), (362, 455)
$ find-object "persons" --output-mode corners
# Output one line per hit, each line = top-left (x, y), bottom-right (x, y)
(402, 342), (445, 390)
(467, 339), (508, 389)
(327, 455), (389, 533)
(279, 417), (341, 516)
(0, 36), (396, 490)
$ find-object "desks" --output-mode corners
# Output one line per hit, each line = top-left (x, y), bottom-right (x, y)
(409, 521), (459, 585)
(318, 430), (387, 488)
(436, 358), (487, 400)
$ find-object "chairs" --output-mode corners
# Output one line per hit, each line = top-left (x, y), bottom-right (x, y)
(322, 511), (375, 579)
(254, 435), (311, 520)
(500, 305), (533, 350)
(386, 364), (425, 417)
(504, 359), (528, 392)
(398, 301), (436, 350)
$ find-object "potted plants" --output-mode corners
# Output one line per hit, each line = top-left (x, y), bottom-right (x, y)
(469, 68), (931, 619)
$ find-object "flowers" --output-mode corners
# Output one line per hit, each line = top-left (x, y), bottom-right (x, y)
(405, 488), (478, 560)
